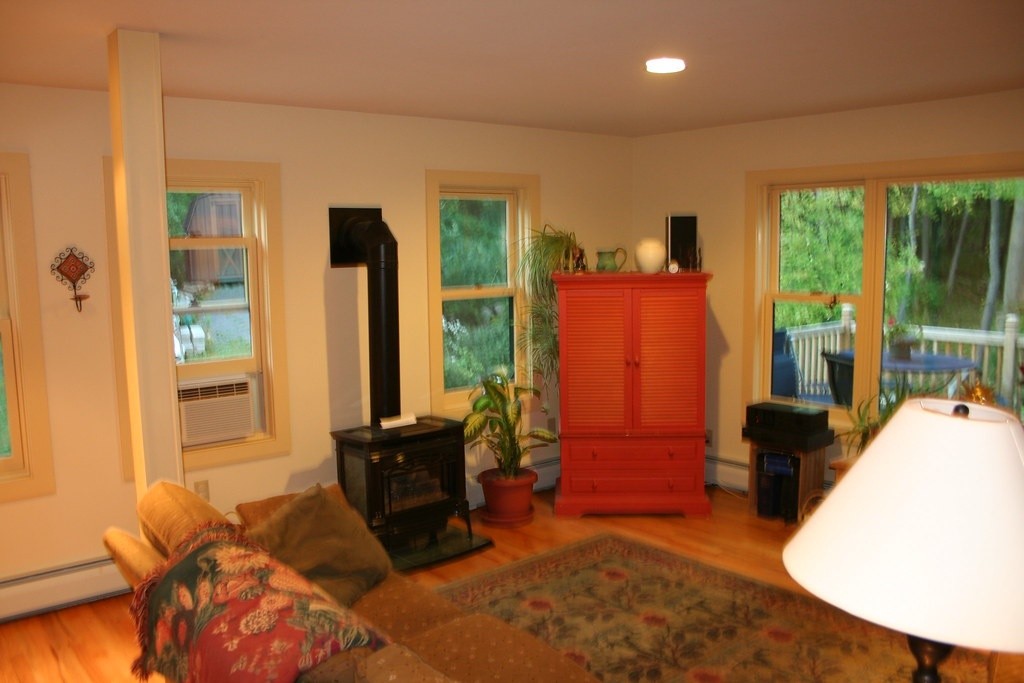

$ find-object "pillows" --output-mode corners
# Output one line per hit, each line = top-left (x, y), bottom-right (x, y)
(237, 481), (366, 531)
(249, 481), (389, 606)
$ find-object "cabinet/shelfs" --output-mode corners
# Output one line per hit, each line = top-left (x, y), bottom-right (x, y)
(552, 273), (713, 520)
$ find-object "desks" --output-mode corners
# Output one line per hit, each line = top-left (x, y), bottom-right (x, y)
(741, 429), (835, 525)
(843, 349), (977, 401)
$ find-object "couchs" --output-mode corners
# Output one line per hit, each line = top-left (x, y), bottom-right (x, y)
(104, 480), (605, 683)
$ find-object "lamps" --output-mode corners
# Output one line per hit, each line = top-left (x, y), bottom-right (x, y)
(782, 397), (1024, 683)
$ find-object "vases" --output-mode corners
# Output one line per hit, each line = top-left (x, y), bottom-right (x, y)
(888, 343), (912, 362)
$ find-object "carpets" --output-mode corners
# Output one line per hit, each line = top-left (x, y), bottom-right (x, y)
(437, 532), (1024, 683)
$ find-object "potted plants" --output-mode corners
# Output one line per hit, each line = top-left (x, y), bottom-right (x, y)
(464, 372), (558, 527)
(507, 225), (587, 414)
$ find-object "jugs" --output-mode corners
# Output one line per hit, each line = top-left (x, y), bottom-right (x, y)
(597, 248), (627, 272)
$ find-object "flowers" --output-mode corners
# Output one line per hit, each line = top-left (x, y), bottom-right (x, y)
(883, 316), (922, 343)
